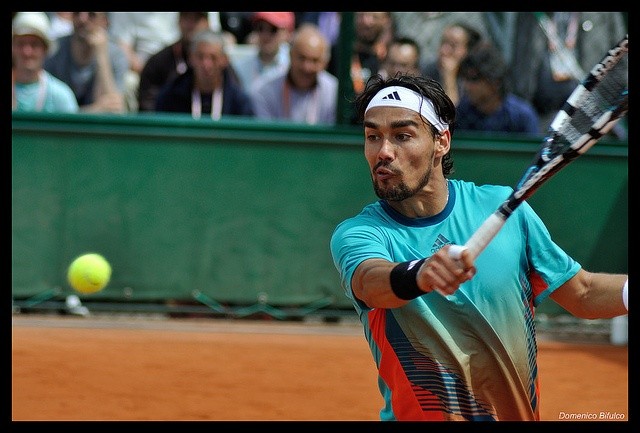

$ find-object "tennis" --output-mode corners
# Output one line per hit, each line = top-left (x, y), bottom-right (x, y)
(68, 253), (111, 293)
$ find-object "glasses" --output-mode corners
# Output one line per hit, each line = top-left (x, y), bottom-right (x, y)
(256, 24), (280, 33)
(72, 12), (104, 19)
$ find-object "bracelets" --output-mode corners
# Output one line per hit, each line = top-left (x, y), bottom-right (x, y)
(387, 259), (429, 300)
(622, 276), (628, 312)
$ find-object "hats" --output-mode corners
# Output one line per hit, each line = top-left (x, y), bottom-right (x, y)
(11, 12), (58, 58)
(254, 12), (296, 32)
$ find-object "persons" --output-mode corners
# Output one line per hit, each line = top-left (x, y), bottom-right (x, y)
(12, 10), (628, 144)
(330, 72), (628, 422)
